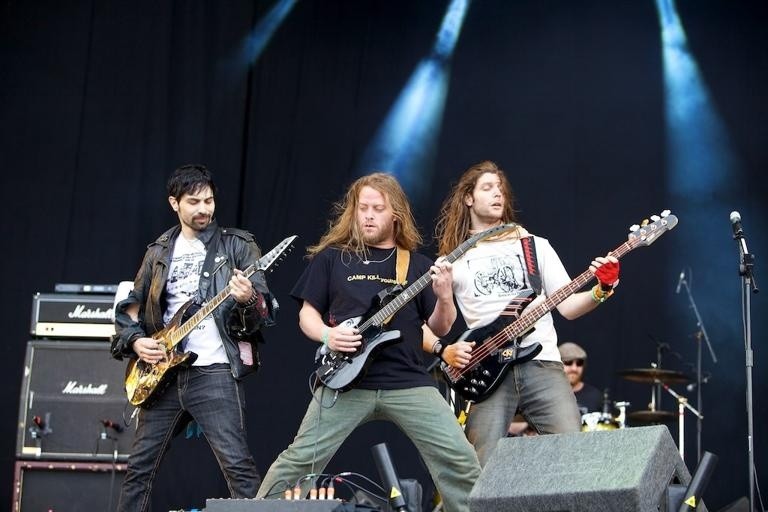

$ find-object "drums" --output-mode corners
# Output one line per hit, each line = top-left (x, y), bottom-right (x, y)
(581, 412), (621, 431)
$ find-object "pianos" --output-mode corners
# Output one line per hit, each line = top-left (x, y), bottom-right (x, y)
(52, 279), (120, 297)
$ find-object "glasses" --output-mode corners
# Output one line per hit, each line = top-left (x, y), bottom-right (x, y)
(563, 360), (583, 367)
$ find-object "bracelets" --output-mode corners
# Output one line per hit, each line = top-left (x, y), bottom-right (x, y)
(591, 284), (615, 305)
(431, 338), (450, 357)
(236, 286), (258, 309)
(320, 326), (332, 344)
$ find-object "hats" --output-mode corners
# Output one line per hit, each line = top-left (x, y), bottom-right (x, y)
(559, 342), (586, 360)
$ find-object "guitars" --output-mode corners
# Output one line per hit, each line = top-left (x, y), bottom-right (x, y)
(439, 210), (679, 402)
(125, 235), (301, 409)
(315, 225), (518, 394)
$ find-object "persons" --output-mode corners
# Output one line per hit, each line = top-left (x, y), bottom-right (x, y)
(112, 160), (282, 512)
(508, 341), (605, 436)
(422, 158), (621, 470)
(254, 169), (485, 512)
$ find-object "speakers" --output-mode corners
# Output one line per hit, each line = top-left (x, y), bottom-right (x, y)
(13, 461), (128, 512)
(16, 339), (132, 462)
(469, 425), (707, 511)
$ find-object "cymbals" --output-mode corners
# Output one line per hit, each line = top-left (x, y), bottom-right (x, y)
(617, 367), (695, 385)
(626, 409), (676, 424)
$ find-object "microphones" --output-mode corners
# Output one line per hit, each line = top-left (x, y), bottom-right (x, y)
(730, 209), (743, 242)
(676, 273), (684, 293)
(686, 377), (707, 391)
